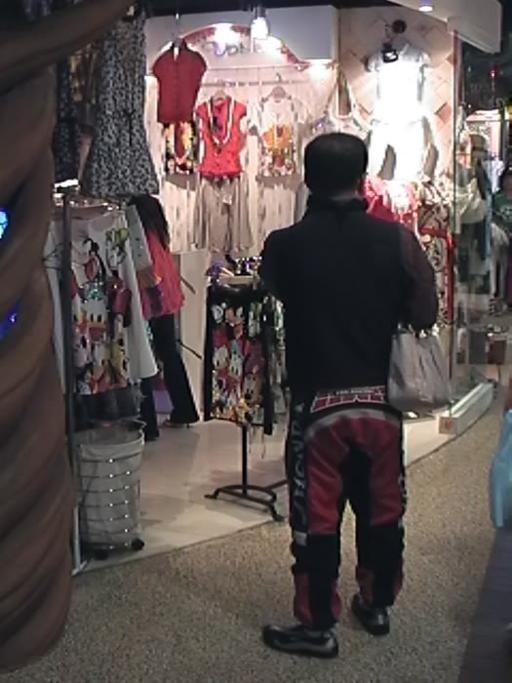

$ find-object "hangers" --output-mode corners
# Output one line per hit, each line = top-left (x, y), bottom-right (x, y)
(379, 22), (410, 53)
(168, 22), (185, 48)
(259, 70), (288, 100)
(207, 79), (231, 100)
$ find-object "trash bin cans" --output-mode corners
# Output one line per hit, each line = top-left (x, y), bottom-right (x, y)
(70, 428), (145, 563)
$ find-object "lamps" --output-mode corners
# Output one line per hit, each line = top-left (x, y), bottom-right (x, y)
(248, 3), (272, 44)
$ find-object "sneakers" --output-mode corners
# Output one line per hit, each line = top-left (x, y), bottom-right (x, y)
(263, 622), (338, 657)
(351, 591), (388, 635)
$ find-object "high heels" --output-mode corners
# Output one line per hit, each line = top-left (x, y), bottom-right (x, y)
(165, 418), (190, 427)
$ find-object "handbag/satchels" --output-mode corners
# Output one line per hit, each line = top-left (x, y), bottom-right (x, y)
(387, 328), (450, 410)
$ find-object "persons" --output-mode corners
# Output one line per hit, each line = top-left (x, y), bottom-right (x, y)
(127, 194), (199, 441)
(492, 166), (512, 307)
(257, 133), (439, 659)
